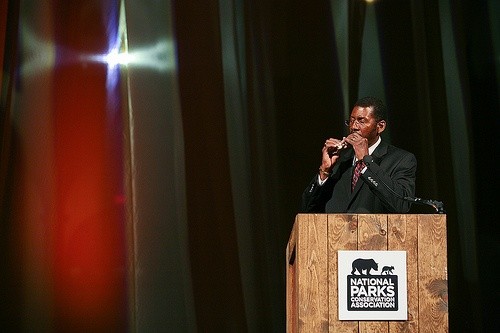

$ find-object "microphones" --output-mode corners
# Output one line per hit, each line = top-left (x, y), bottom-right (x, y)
(355, 158), (439, 214)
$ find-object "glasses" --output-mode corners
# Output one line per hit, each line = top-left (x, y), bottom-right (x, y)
(344, 119), (379, 127)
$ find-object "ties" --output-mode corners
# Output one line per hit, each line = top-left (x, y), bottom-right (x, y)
(352, 160), (367, 192)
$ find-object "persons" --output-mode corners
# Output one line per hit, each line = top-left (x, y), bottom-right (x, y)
(301, 98), (417, 213)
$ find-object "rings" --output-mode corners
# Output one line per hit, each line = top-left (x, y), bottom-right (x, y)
(351, 137), (357, 141)
